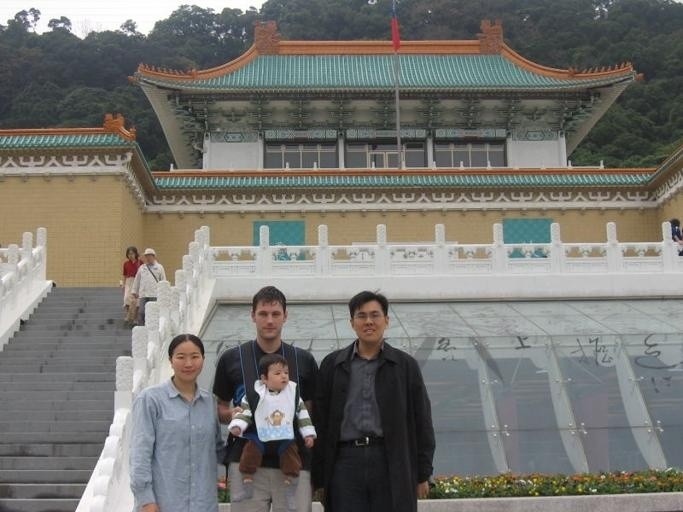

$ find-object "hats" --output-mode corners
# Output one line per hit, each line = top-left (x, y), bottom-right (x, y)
(144, 248), (155, 255)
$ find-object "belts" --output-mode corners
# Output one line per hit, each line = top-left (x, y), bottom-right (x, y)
(339, 436), (382, 448)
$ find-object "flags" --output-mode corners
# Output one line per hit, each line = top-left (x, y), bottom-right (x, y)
(391, 6), (402, 55)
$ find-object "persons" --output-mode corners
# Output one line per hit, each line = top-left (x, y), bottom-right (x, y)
(224, 352), (319, 477)
(130, 247), (167, 327)
(671, 219), (683, 256)
(304, 286), (436, 512)
(209, 285), (321, 512)
(126, 333), (226, 512)
(120, 246), (144, 329)
